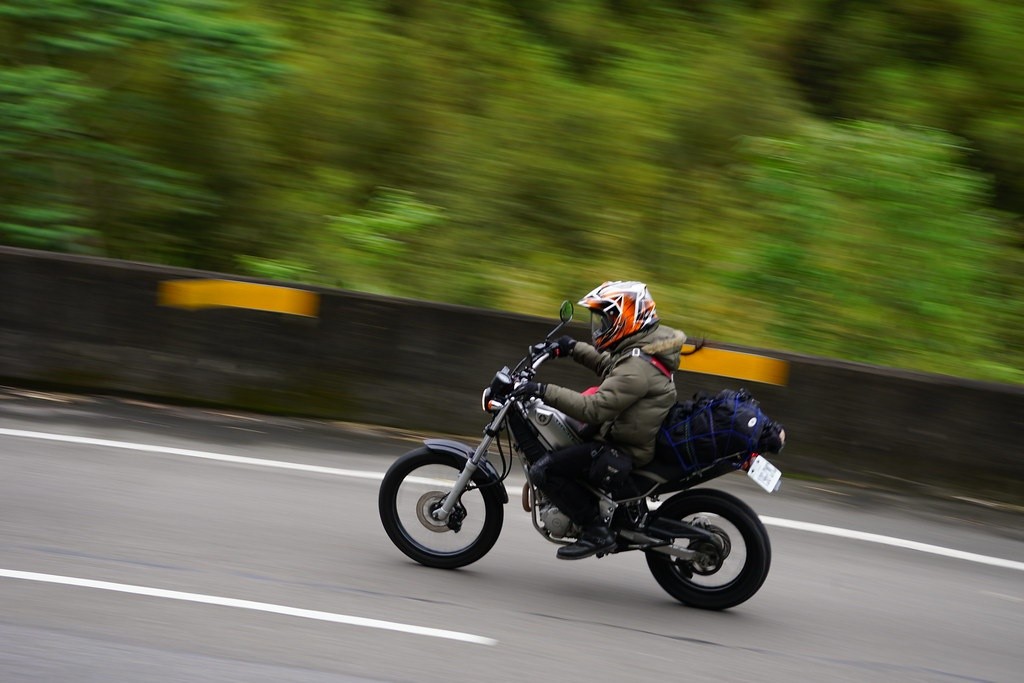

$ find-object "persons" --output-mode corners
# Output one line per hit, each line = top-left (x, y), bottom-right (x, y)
(510, 279), (689, 561)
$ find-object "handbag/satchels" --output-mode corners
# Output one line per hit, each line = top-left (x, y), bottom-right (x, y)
(654, 388), (769, 485)
(588, 443), (633, 491)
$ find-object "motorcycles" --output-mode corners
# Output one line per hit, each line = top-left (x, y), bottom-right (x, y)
(378, 300), (786, 611)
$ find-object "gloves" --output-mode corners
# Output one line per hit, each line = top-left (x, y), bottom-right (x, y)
(557, 334), (577, 350)
(512, 381), (546, 402)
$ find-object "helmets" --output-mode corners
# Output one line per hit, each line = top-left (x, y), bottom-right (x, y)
(577, 281), (659, 354)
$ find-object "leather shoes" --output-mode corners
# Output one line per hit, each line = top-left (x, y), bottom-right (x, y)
(555, 516), (617, 560)
